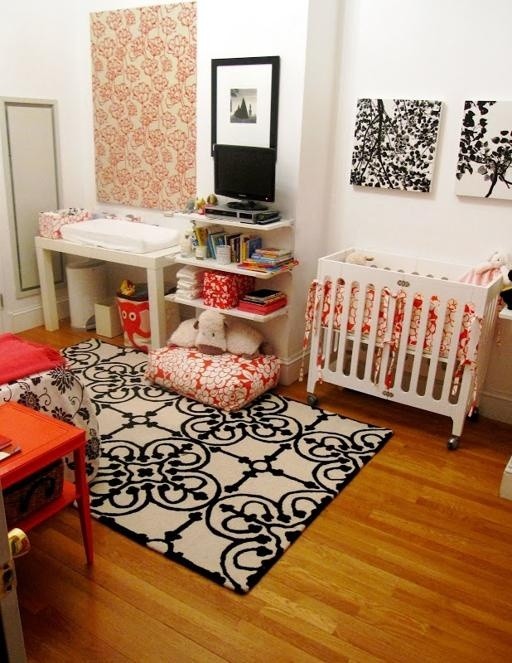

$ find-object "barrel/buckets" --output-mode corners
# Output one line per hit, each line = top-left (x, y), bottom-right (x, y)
(65, 260), (107, 330)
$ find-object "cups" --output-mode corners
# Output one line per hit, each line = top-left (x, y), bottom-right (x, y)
(195, 245), (208, 260)
(216, 244), (232, 266)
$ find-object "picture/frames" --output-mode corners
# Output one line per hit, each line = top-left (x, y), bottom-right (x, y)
(210, 55), (281, 157)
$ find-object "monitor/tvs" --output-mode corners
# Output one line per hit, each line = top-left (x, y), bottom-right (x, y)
(214, 144), (276, 210)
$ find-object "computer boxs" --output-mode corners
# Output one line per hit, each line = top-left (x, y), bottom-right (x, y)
(205, 203), (280, 225)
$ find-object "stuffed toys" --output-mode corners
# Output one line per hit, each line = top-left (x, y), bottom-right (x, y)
(167, 309), (266, 360)
(455, 249), (508, 286)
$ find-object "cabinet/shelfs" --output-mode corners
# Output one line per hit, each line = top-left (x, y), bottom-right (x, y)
(0, 400), (92, 577)
(163, 210), (294, 323)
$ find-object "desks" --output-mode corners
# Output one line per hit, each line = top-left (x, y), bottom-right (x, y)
(34, 236), (179, 349)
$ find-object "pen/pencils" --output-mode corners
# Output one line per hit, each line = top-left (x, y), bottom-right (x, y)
(194, 226), (202, 246)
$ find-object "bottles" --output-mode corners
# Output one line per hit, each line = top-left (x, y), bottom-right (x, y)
(180, 234), (192, 258)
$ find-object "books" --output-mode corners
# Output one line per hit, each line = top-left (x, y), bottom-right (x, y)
(237, 287), (288, 316)
(191, 224), (300, 274)
(1, 432), (22, 461)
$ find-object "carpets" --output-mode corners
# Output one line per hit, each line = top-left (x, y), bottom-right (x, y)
(58, 338), (395, 593)
